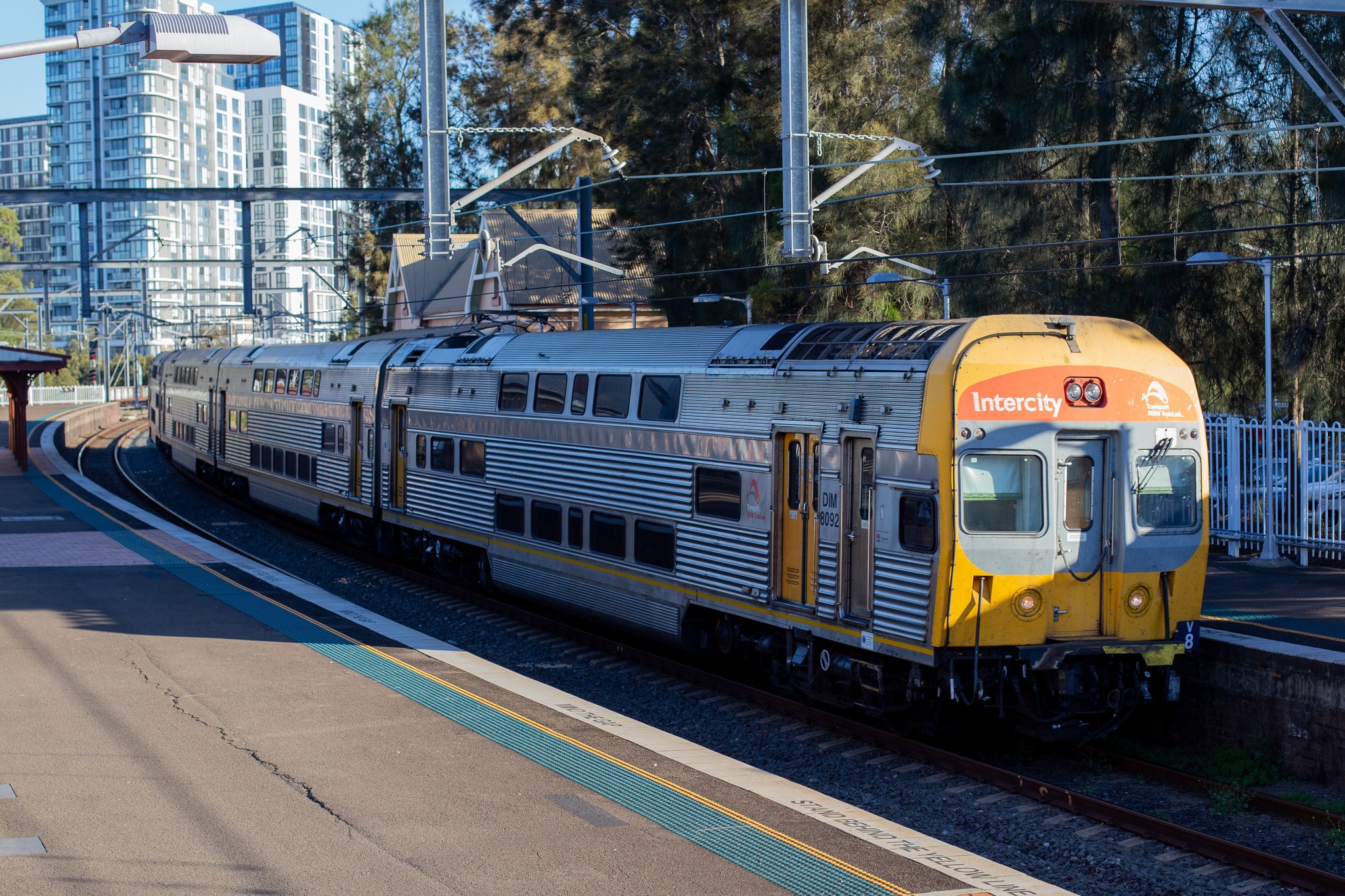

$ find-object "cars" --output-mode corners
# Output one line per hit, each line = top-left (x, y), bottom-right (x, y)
(1208, 457), (1345, 533)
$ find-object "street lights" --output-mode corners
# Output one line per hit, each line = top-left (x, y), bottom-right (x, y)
(694, 294), (752, 323)
(865, 272), (950, 319)
(579, 296), (636, 329)
(1185, 252), (1295, 568)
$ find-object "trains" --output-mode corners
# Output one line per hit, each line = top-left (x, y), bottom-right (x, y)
(148, 315), (1211, 731)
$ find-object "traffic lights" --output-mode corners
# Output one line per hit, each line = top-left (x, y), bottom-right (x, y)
(90, 349), (98, 362)
(88, 368), (97, 382)
